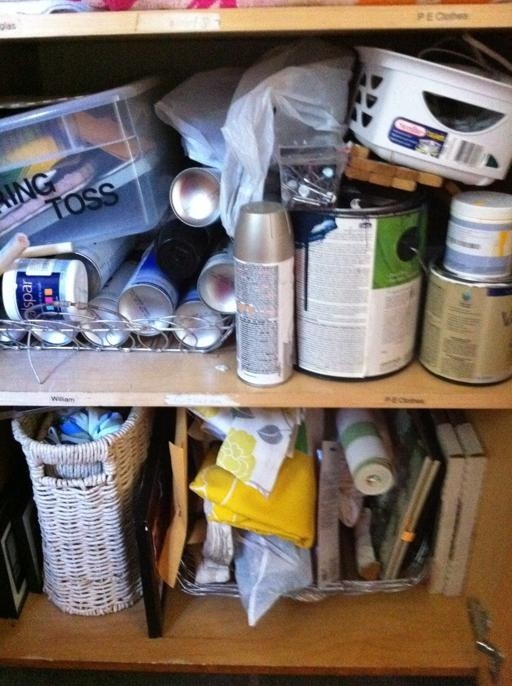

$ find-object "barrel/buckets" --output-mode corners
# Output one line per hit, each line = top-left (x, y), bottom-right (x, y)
(289, 187), (430, 382)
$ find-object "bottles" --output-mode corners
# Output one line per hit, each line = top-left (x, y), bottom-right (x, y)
(0, 165), (292, 349)
(231, 199), (293, 387)
(440, 188), (511, 281)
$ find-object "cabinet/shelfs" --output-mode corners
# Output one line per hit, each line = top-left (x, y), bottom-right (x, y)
(0, 1), (512, 686)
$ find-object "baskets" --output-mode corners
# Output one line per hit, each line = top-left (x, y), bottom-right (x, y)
(350, 42), (512, 187)
(12, 405), (153, 618)
